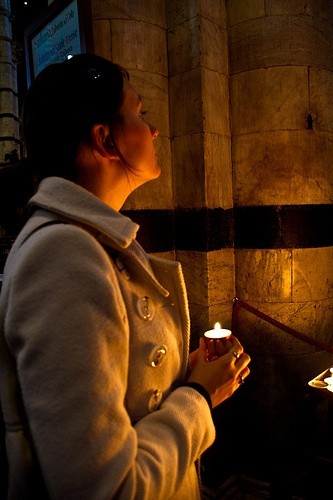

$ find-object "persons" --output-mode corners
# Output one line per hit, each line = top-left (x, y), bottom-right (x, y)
(0, 53), (252, 500)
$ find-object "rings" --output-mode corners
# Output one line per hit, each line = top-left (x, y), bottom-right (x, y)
(239, 373), (244, 384)
(229, 349), (239, 358)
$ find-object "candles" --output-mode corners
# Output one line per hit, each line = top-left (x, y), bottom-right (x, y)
(204, 321), (232, 362)
